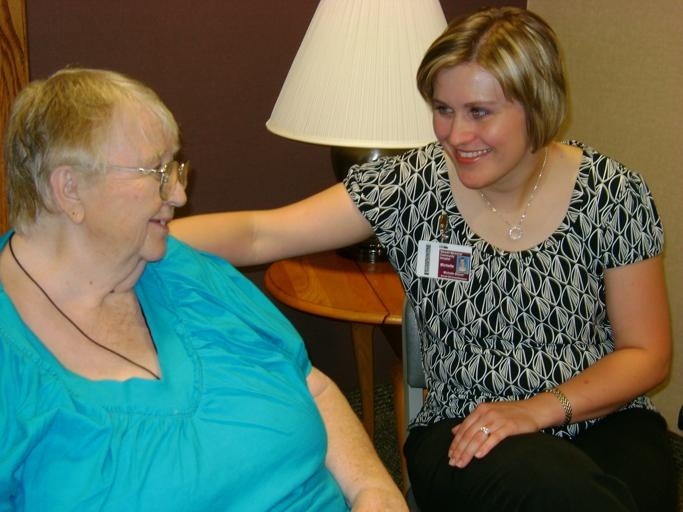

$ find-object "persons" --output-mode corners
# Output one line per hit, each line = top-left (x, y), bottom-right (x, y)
(168, 6), (682, 512)
(0, 66), (412, 512)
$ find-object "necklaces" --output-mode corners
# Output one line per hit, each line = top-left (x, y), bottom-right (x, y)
(476, 144), (549, 244)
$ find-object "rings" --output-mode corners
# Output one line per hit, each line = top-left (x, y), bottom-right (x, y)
(479, 426), (492, 437)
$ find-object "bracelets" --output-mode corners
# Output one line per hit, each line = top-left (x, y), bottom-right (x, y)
(545, 386), (573, 429)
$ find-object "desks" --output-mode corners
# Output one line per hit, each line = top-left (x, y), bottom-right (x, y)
(264, 251), (405, 442)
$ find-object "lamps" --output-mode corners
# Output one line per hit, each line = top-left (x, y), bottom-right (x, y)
(265, 0), (449, 264)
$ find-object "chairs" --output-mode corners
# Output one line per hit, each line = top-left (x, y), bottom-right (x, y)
(403, 302), (427, 512)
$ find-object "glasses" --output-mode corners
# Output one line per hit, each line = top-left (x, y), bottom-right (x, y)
(107, 161), (190, 199)
